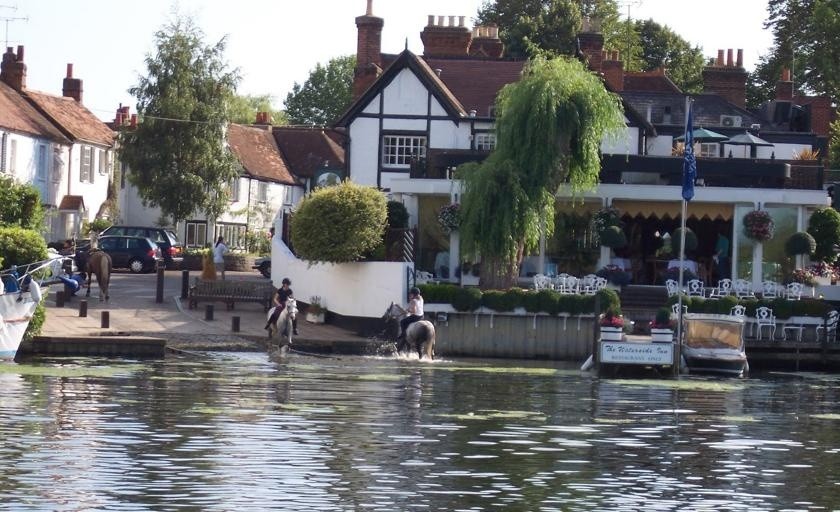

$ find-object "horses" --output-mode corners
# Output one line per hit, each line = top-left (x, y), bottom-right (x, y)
(73, 247), (113, 304)
(380, 301), (436, 361)
(267, 296), (298, 353)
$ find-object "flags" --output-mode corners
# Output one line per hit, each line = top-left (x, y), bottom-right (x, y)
(680, 98), (698, 201)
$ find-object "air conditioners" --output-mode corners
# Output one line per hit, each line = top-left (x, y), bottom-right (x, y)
(720, 115), (742, 127)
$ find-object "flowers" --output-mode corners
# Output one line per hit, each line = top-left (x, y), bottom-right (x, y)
(648, 319), (673, 329)
(742, 210), (776, 242)
(435, 201), (462, 234)
(600, 316), (624, 329)
(597, 265), (633, 285)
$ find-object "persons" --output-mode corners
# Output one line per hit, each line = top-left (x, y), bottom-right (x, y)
(262, 277), (299, 335)
(396, 286), (424, 340)
(68, 271), (89, 297)
(58, 238), (74, 273)
(712, 230), (731, 286)
(4, 264), (19, 292)
(211, 236), (231, 280)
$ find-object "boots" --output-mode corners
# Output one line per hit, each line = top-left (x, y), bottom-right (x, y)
(264, 314), (274, 329)
(293, 318), (299, 335)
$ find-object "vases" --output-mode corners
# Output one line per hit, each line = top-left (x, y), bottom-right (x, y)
(651, 328), (674, 343)
(601, 326), (622, 341)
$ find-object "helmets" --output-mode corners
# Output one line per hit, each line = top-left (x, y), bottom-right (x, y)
(282, 279), (291, 285)
(411, 287), (420, 294)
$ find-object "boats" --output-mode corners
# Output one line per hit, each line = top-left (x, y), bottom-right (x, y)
(676, 312), (749, 376)
(0, 253), (78, 363)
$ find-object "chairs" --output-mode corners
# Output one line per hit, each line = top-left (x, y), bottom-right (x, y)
(533, 272), (608, 295)
(666, 278), (840, 342)
(697, 262), (712, 287)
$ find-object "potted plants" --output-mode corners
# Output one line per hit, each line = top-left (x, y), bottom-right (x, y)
(304, 297), (327, 324)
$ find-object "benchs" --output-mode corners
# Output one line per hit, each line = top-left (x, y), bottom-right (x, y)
(187, 279), (278, 311)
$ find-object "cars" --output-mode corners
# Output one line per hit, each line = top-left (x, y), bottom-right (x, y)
(58, 225), (185, 275)
